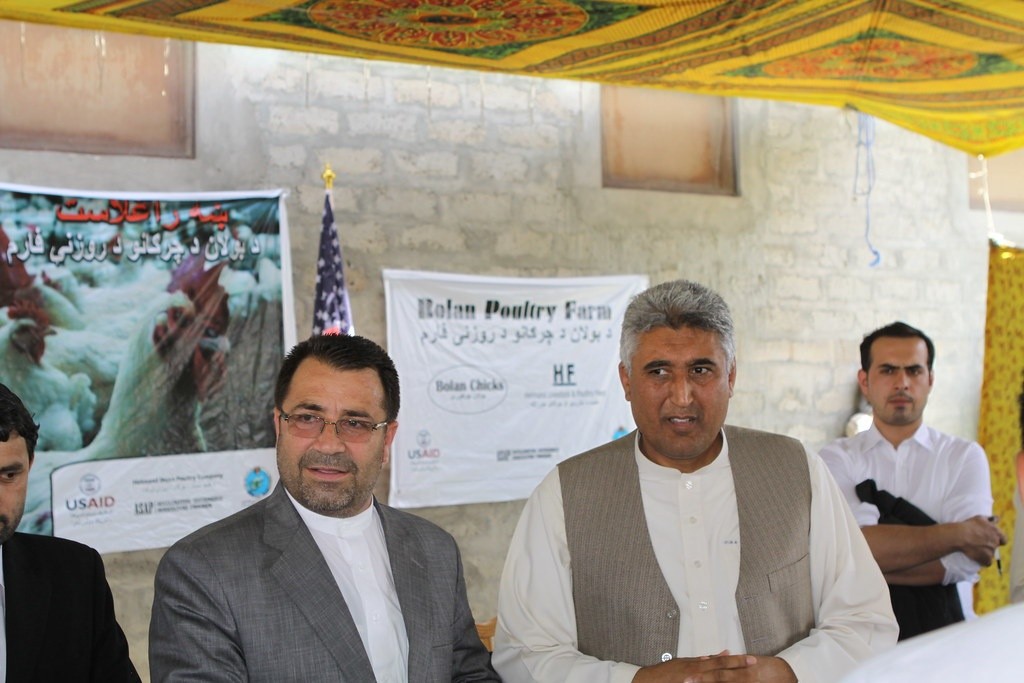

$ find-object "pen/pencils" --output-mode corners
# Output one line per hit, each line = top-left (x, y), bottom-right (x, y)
(988, 517), (1003, 577)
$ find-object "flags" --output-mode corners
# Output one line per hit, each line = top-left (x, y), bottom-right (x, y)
(311, 192), (356, 336)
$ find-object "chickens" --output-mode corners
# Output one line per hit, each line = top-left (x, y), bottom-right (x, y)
(1, 208), (283, 540)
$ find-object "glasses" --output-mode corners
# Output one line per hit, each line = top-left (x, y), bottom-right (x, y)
(278, 405), (390, 445)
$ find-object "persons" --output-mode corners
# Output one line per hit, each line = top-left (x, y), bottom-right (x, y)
(0, 383), (143, 683)
(149, 335), (498, 683)
(490, 279), (900, 683)
(816, 321), (1007, 642)
